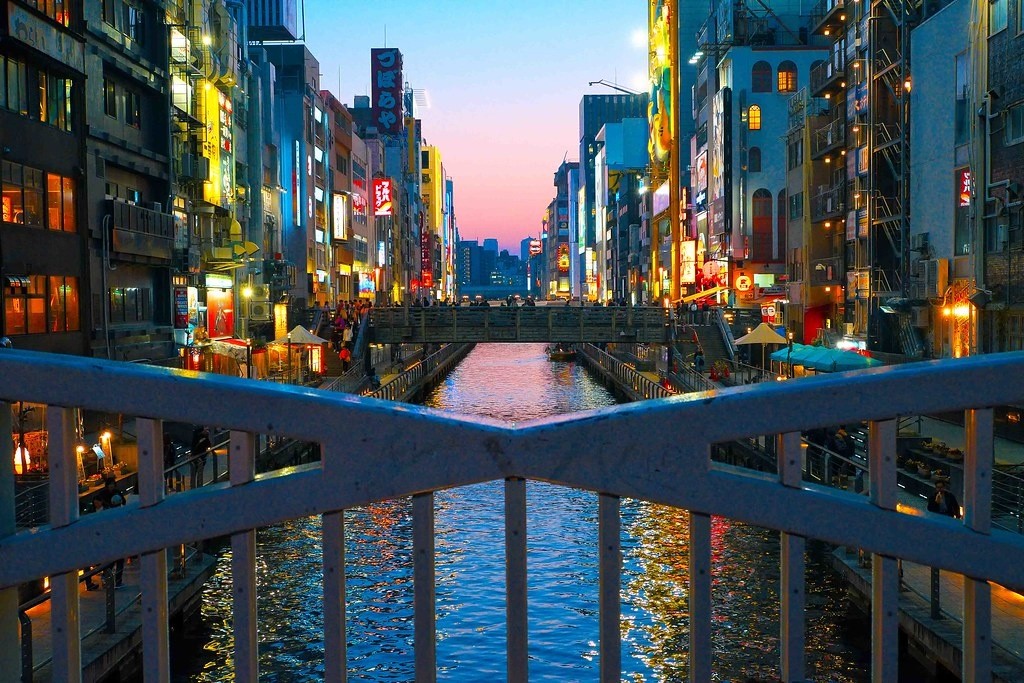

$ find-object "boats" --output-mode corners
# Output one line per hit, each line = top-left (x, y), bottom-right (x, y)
(546, 345), (575, 362)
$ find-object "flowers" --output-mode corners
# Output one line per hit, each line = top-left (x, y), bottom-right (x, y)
(78, 460), (130, 487)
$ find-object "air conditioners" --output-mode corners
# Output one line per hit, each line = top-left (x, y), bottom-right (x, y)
(253, 302), (270, 320)
(912, 257), (918, 275)
(911, 306), (930, 326)
(916, 259), (928, 299)
(913, 232), (925, 251)
(929, 258), (950, 298)
(910, 283), (918, 299)
(253, 284), (271, 302)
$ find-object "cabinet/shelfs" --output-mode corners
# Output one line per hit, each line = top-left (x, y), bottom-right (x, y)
(897, 446), (964, 507)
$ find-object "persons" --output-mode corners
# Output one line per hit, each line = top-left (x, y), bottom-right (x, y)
(469, 299), (490, 306)
(634, 300), (640, 312)
(83, 477), (126, 589)
(190, 427), (211, 490)
(413, 296), (459, 307)
(694, 348), (705, 374)
(374, 300), (404, 308)
(926, 479), (960, 519)
(163, 432), (176, 493)
(507, 295), (535, 307)
(805, 424), (855, 490)
(339, 346), (351, 374)
(594, 298), (627, 311)
(324, 301), (330, 321)
(334, 298), (372, 353)
(675, 299), (709, 326)
(313, 301), (320, 309)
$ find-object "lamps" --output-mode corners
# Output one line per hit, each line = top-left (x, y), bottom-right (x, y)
(854, 57), (881, 68)
(266, 181), (288, 194)
(824, 89), (832, 99)
(824, 154), (832, 164)
(688, 38), (733, 64)
(638, 175), (668, 195)
(852, 122), (870, 132)
(968, 290), (993, 311)
(824, 24), (842, 36)
(853, 189), (881, 199)
(814, 263), (826, 271)
(825, 220), (830, 228)
(841, 79), (846, 88)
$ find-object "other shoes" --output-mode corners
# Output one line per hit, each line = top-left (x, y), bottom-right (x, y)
(169, 487), (176, 491)
(87, 584), (100, 591)
(115, 584), (127, 588)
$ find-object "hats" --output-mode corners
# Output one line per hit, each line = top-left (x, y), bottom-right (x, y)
(111, 494), (122, 505)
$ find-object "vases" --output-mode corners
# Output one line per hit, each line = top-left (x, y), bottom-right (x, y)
(79, 484), (89, 495)
(116, 470), (127, 476)
(87, 478), (103, 487)
(104, 471), (115, 480)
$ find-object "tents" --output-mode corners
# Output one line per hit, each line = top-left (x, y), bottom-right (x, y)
(771, 343), (886, 380)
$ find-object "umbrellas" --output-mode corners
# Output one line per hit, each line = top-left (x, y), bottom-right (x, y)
(272, 324), (329, 368)
(733, 323), (795, 369)
